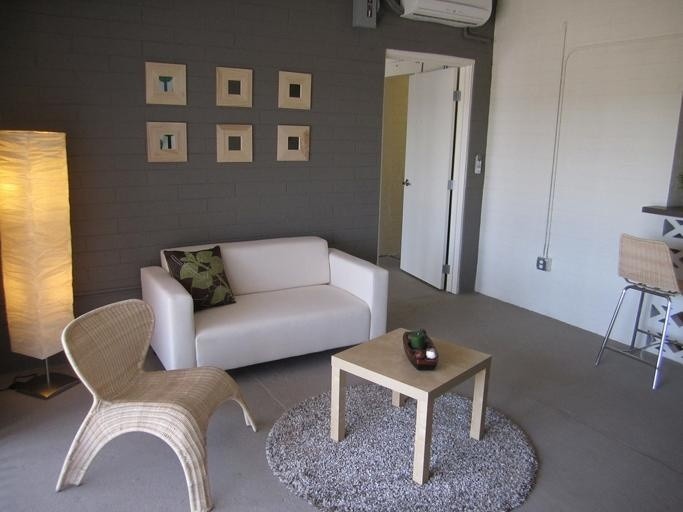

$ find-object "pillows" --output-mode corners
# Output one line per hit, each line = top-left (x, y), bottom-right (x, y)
(153, 245), (232, 309)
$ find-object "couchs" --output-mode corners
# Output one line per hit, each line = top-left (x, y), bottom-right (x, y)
(141, 234), (388, 368)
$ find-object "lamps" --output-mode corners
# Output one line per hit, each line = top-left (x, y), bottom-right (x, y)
(0, 129), (81, 399)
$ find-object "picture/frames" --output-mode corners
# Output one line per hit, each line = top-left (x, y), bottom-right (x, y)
(215, 124), (252, 163)
(144, 61), (186, 105)
(144, 121), (188, 163)
(276, 69), (312, 110)
(214, 66), (253, 109)
(275, 124), (310, 163)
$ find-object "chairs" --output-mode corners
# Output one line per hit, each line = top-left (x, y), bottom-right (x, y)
(50, 299), (259, 512)
(590, 231), (682, 391)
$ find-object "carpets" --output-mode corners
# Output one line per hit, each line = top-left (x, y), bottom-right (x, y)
(269, 387), (541, 512)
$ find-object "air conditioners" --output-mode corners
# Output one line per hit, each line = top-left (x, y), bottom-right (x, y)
(386, 1), (492, 30)
